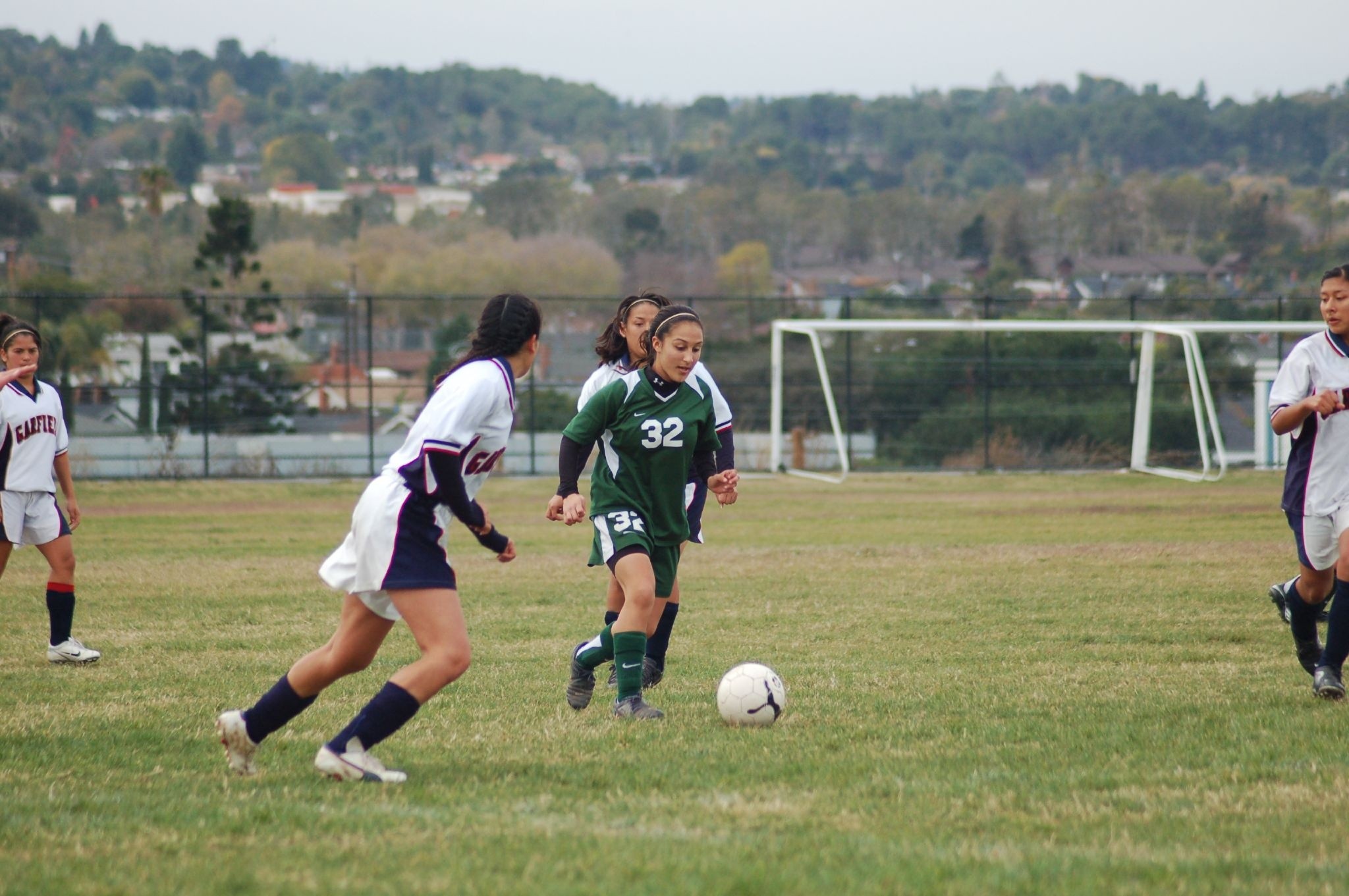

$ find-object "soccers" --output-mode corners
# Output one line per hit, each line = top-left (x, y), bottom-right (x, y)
(715, 663), (787, 727)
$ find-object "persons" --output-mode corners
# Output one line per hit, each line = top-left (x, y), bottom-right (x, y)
(560, 305), (740, 724)
(211, 293), (541, 781)
(544, 286), (737, 691)
(1269, 264), (1349, 698)
(0, 313), (100, 664)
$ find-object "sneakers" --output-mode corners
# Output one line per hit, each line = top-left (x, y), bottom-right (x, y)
(47, 634), (100, 666)
(639, 659), (665, 690)
(1311, 665), (1346, 699)
(606, 661), (618, 689)
(614, 697), (663, 719)
(214, 710), (260, 779)
(314, 737), (407, 785)
(566, 641), (597, 708)
(1268, 583), (1292, 624)
(1284, 600), (1324, 674)
